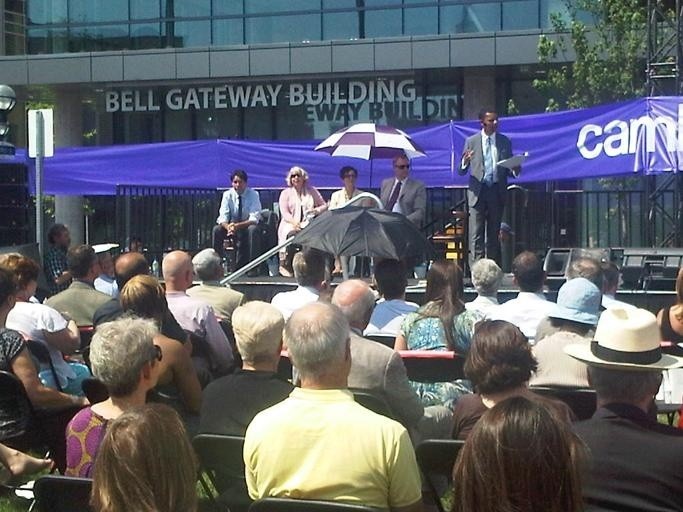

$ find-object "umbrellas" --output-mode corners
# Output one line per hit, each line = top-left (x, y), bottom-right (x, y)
(289, 201), (442, 286)
(311, 118), (430, 193)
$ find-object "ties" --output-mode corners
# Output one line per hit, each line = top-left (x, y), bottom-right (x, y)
(484, 137), (492, 188)
(384, 182), (401, 212)
(237, 195), (243, 222)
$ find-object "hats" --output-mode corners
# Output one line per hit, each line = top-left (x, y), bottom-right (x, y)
(565, 306), (683, 372)
(544, 278), (604, 324)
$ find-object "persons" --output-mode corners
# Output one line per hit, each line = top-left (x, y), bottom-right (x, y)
(276, 165), (330, 277)
(327, 166), (371, 277)
(211, 169), (263, 279)
(456, 107), (524, 289)
(1, 221), (683, 512)
(373, 152), (427, 277)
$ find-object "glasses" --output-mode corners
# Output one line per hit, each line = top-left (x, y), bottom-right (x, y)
(396, 164), (409, 169)
(291, 174), (299, 179)
(219, 258), (226, 265)
(151, 345), (162, 362)
